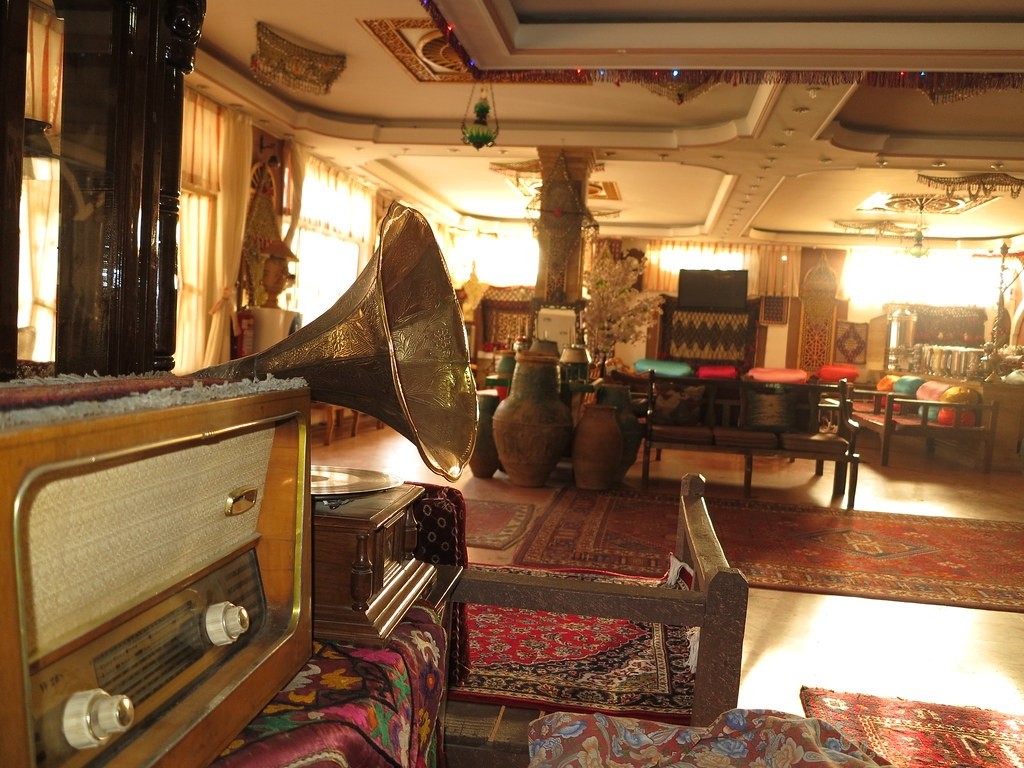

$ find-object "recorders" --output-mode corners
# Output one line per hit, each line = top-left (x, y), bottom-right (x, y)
(0, 384), (311, 768)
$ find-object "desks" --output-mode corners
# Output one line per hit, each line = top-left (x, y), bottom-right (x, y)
(205, 559), (461, 768)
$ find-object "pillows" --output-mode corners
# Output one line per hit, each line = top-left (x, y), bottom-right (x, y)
(870, 373), (979, 426)
(633, 358), (697, 379)
(652, 378), (705, 426)
(816, 365), (861, 385)
(695, 366), (738, 381)
(737, 383), (801, 435)
(744, 366), (809, 384)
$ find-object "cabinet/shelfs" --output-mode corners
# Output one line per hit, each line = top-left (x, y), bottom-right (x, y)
(661, 306), (758, 375)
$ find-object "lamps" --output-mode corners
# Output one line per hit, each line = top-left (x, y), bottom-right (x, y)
(558, 346), (588, 383)
(461, 78), (500, 152)
(904, 211), (931, 259)
(885, 195), (964, 215)
(834, 192), (927, 246)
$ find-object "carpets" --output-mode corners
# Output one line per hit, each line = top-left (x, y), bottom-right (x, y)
(511, 483), (1024, 613)
(461, 498), (536, 550)
(799, 685), (1024, 768)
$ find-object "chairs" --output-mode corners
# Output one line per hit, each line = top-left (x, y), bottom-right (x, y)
(311, 401), (359, 446)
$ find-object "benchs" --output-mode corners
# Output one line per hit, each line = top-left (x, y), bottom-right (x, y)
(642, 368), (861, 507)
(817, 383), (998, 473)
(404, 472), (746, 768)
